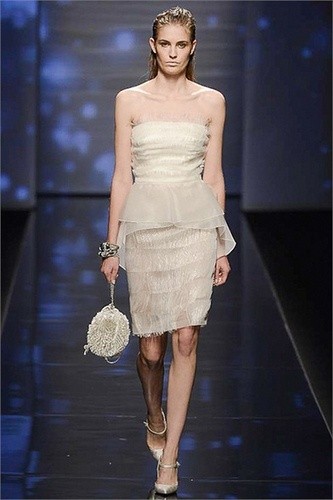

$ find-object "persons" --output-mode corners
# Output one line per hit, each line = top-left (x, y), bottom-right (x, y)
(97, 7), (236, 495)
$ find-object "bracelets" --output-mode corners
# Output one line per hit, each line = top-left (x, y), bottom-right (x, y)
(97, 242), (119, 257)
